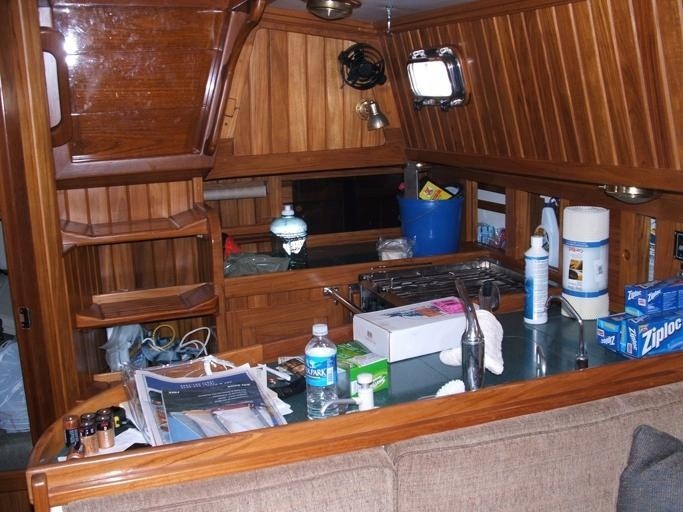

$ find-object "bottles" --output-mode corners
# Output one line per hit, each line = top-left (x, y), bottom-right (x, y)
(304, 323), (339, 420)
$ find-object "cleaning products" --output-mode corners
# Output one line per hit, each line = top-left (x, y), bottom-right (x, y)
(539, 195), (560, 270)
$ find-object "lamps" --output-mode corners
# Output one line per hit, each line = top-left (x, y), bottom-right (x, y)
(356, 98), (389, 132)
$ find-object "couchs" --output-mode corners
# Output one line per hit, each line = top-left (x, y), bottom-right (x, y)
(59, 379), (683, 512)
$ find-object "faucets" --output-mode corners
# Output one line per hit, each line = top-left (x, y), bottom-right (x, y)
(454, 278), (486, 391)
(543, 293), (590, 366)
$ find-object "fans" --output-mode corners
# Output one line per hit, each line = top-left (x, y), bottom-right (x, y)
(337, 41), (386, 92)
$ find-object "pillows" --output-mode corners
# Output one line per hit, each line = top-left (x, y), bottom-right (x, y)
(616, 425), (681, 511)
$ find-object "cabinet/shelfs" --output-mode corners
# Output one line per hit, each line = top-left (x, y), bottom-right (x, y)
(217, 162), (682, 357)
